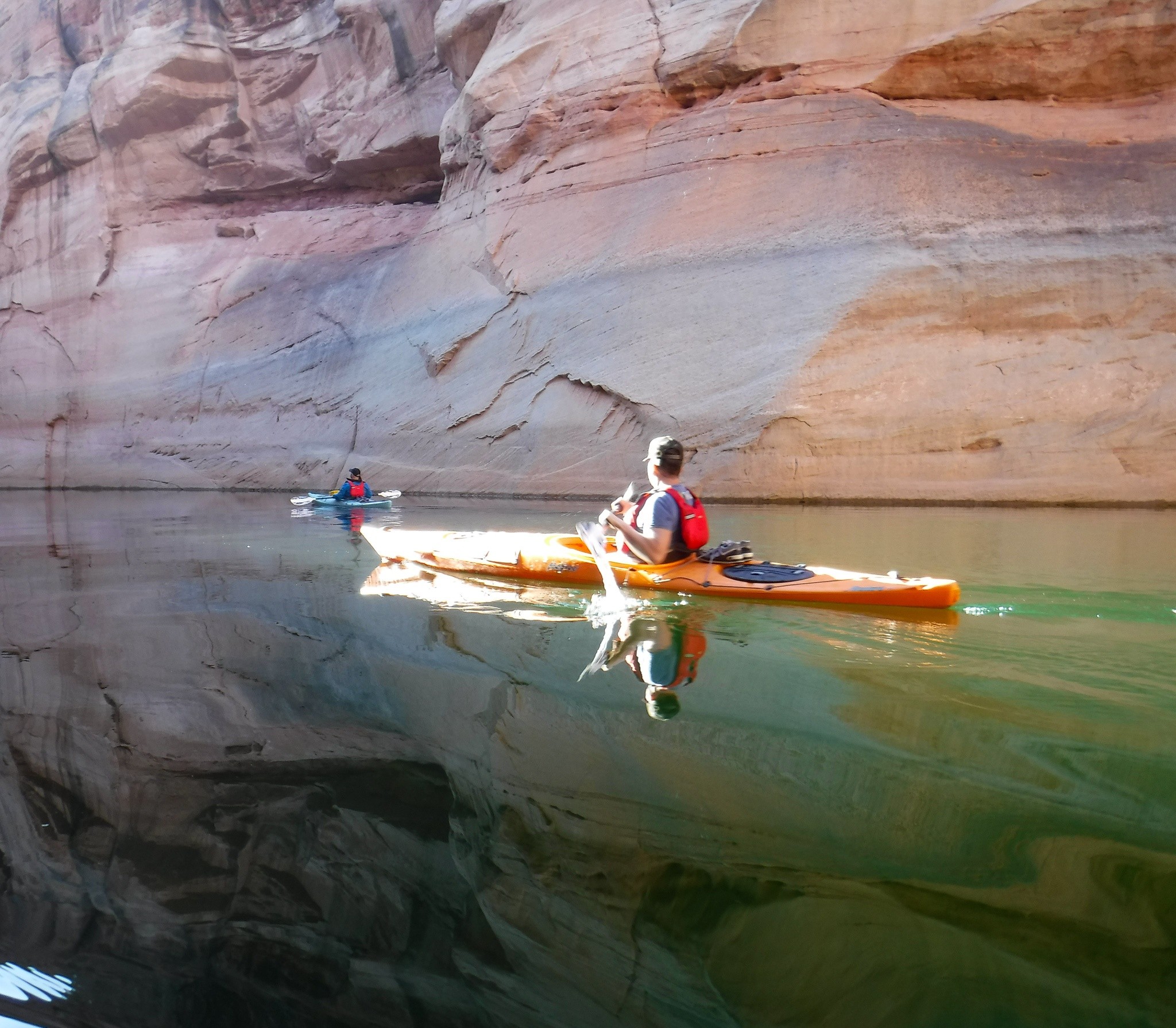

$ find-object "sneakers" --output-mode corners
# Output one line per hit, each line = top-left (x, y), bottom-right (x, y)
(726, 540), (753, 562)
(696, 540), (745, 564)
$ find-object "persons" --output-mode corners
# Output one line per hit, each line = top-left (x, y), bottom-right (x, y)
(597, 592), (708, 721)
(596, 435), (705, 565)
(332, 467), (372, 503)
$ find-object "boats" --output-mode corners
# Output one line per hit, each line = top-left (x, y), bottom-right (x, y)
(360, 521), (963, 610)
(307, 492), (393, 510)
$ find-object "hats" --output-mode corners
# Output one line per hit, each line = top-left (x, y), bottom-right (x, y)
(349, 468), (360, 477)
(642, 436), (683, 463)
(641, 693), (680, 721)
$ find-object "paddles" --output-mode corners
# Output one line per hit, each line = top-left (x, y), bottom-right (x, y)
(290, 489), (402, 506)
(575, 480), (636, 553)
